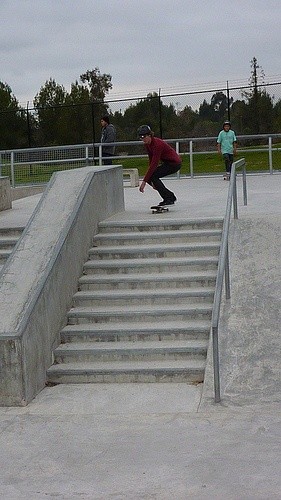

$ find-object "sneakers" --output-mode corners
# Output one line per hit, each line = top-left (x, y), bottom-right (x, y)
(226, 172), (230, 175)
(159, 196), (177, 205)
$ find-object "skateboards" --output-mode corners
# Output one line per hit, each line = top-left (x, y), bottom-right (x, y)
(223, 174), (237, 181)
(151, 202), (175, 214)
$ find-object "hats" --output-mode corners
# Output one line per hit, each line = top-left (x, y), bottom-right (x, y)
(102, 116), (109, 123)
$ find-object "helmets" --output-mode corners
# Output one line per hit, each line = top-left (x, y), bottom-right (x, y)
(137, 125), (151, 138)
(223, 121), (231, 129)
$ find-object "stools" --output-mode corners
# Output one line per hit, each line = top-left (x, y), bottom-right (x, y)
(123, 168), (139, 188)
